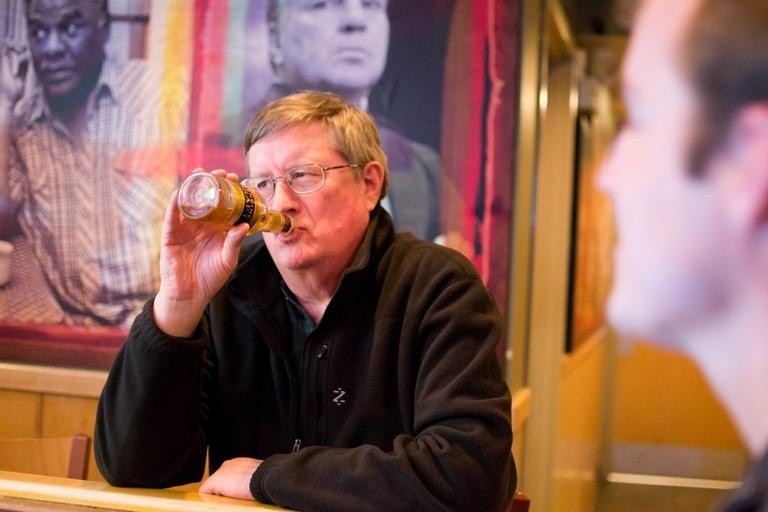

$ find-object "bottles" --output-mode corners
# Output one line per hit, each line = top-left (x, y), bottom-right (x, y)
(177, 172), (295, 237)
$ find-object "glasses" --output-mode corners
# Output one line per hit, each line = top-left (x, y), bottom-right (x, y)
(237, 161), (366, 204)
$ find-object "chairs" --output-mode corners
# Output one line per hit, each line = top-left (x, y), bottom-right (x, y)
(0, 431), (92, 483)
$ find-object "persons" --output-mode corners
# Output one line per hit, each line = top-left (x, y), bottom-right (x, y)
(205, 1), (439, 246)
(94, 90), (516, 512)
(596, 0), (768, 512)
(0, 0), (167, 323)
